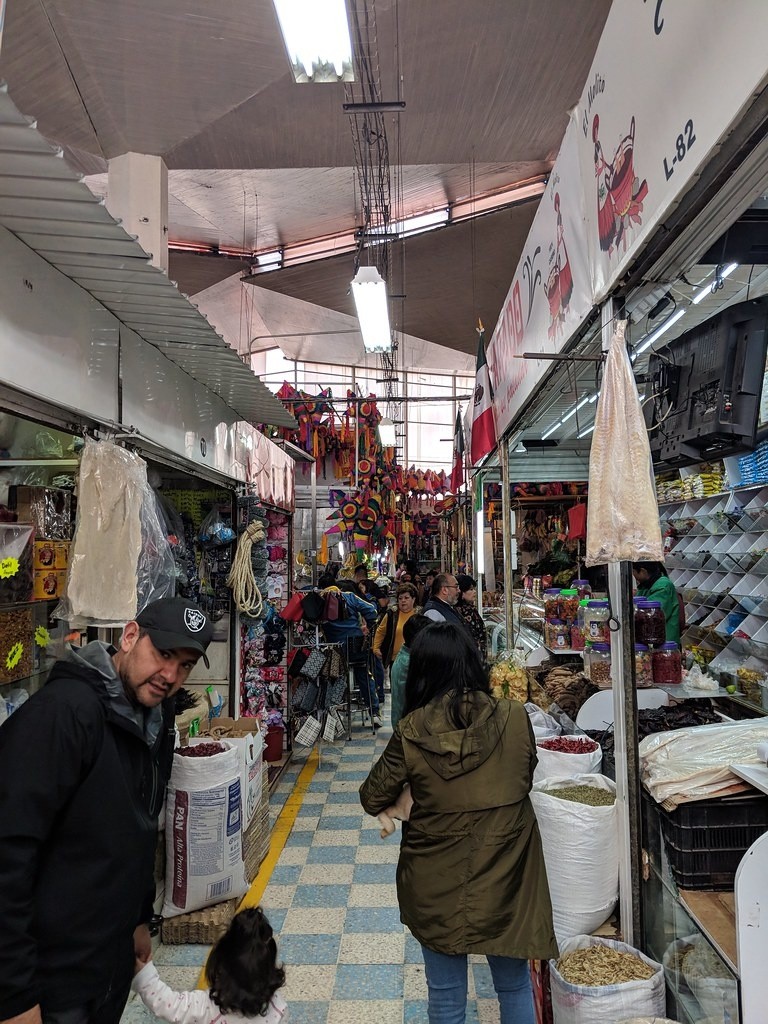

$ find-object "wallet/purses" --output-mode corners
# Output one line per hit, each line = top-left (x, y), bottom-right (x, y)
(288, 645), (347, 748)
(280, 591), (349, 623)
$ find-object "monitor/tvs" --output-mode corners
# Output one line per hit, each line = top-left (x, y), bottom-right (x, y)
(643, 292), (768, 473)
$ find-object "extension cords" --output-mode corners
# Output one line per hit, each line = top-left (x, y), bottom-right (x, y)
(510, 511), (516, 535)
(511, 539), (517, 570)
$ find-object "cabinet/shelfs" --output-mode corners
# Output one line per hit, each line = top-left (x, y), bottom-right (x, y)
(2, 425), (768, 1024)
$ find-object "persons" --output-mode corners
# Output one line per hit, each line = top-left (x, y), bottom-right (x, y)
(358, 622), (559, 1024)
(336, 555), (443, 705)
(421, 573), (460, 624)
(631, 558), (679, 645)
(318, 573), (382, 728)
(453, 576), (487, 657)
(134, 908), (287, 1024)
(0, 598), (215, 1024)
(391, 613), (433, 731)
(372, 583), (424, 672)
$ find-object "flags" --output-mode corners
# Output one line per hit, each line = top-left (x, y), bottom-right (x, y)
(447, 409), (463, 493)
(470, 337), (497, 465)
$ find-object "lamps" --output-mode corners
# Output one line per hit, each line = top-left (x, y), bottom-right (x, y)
(514, 442), (526, 453)
(541, 262), (738, 440)
(350, 266), (394, 354)
(271, 0), (356, 84)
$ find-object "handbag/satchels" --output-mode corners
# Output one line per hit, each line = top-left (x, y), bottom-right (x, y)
(377, 782), (414, 838)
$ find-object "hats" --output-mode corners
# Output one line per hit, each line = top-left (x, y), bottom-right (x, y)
(135, 597), (216, 670)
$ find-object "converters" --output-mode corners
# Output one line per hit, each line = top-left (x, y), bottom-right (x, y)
(648, 297), (670, 320)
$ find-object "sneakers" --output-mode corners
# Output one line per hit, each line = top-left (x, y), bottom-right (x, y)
(372, 715), (383, 726)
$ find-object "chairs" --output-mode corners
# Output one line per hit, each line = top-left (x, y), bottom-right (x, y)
(321, 633), (375, 741)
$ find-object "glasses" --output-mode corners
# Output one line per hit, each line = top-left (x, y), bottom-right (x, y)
(445, 585), (460, 590)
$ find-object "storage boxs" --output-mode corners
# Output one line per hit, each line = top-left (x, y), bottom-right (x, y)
(34, 571), (67, 599)
(17, 486), (71, 538)
(184, 717), (262, 832)
(610, 759), (768, 891)
(35, 541), (69, 570)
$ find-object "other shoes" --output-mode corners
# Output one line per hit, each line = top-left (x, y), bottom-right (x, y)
(380, 697), (384, 703)
